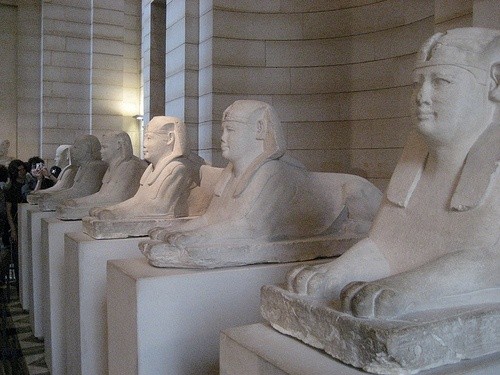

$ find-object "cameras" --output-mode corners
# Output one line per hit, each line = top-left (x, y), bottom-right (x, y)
(36, 163), (43, 170)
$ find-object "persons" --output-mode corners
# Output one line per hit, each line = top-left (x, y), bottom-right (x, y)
(87, 115), (228, 221)
(37, 134), (111, 201)
(147, 97), (387, 248)
(0, 156), (63, 299)
(61, 128), (153, 208)
(282, 27), (500, 322)
(29, 143), (82, 194)
